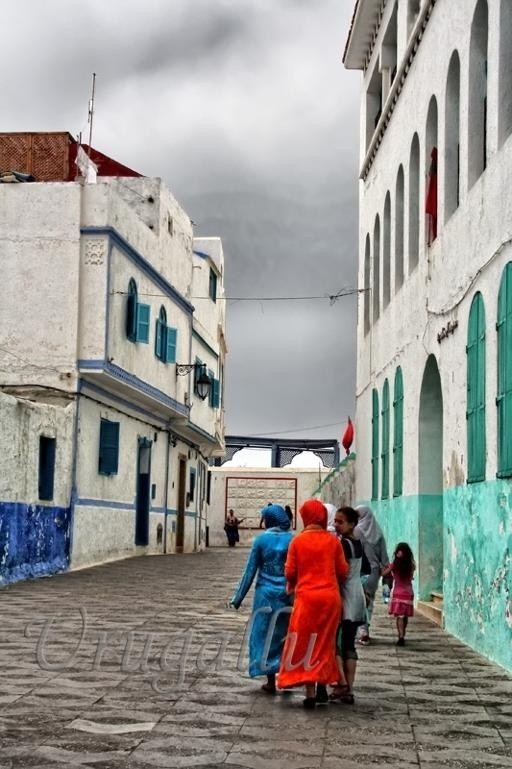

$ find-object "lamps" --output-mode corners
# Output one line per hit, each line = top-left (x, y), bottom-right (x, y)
(176, 361), (212, 399)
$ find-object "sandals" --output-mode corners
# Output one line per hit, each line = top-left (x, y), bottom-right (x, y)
(302, 681), (354, 709)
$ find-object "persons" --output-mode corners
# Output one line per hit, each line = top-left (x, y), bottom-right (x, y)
(277, 497), (351, 708)
(354, 504), (391, 646)
(381, 542), (418, 647)
(284, 505), (294, 531)
(327, 506), (375, 705)
(323, 502), (342, 537)
(258, 502), (273, 529)
(230, 504), (298, 694)
(224, 508), (244, 547)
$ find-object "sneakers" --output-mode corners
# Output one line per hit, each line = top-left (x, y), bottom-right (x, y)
(261, 685), (292, 695)
(397, 638), (404, 646)
(358, 635), (370, 646)
